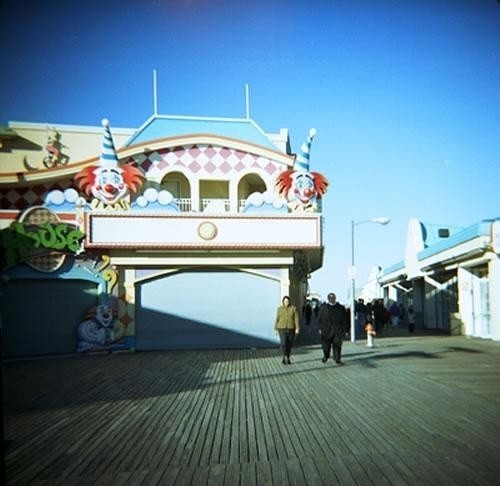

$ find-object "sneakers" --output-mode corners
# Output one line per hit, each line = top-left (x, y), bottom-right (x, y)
(283, 359), (291, 364)
(321, 356), (328, 362)
(335, 361), (344, 366)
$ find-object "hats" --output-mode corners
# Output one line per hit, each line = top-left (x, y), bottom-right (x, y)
(328, 293), (336, 303)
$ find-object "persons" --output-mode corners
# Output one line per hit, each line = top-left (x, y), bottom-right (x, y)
(302, 292), (416, 365)
(273, 295), (300, 365)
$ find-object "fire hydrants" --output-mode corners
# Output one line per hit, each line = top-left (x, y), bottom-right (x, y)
(365, 324), (376, 348)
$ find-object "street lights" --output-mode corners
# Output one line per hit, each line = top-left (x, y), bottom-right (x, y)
(351, 215), (391, 342)
